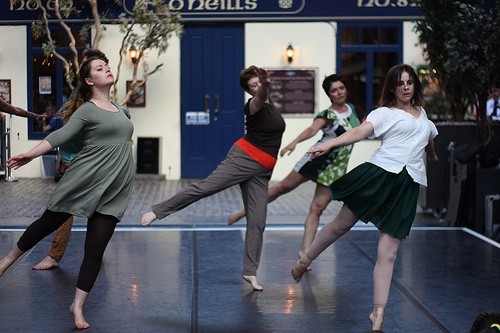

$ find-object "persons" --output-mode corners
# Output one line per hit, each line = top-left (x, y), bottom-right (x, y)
(1, 55), (136, 329)
(228, 73), (361, 271)
(1, 98), (46, 130)
(143, 65), (286, 291)
(32, 51), (108, 270)
(291, 64), (440, 332)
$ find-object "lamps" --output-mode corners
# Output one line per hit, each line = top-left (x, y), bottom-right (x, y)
(286, 44), (295, 63)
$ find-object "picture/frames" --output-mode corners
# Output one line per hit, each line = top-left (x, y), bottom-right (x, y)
(126, 80), (147, 107)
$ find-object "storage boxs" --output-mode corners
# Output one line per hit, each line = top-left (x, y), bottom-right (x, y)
(483, 194), (500, 238)
